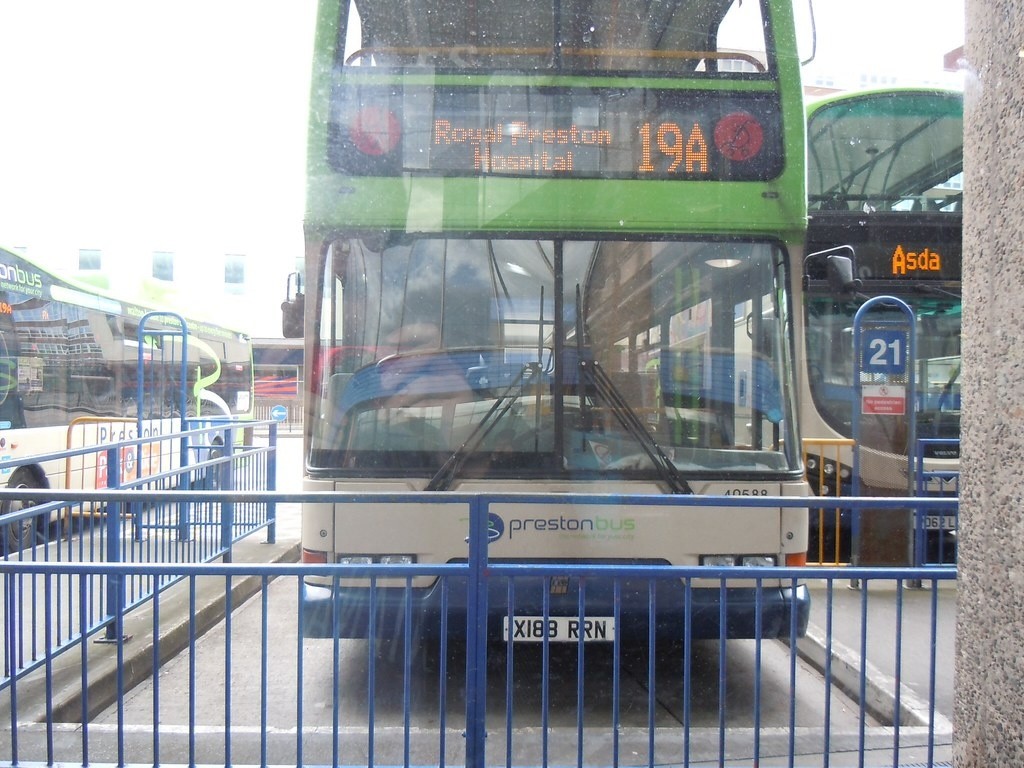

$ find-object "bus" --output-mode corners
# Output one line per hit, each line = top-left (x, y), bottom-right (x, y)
(0, 246), (255, 554)
(280, 0), (813, 642)
(649, 86), (963, 532)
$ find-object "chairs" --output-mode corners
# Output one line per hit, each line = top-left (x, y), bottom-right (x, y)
(910, 199), (941, 212)
(818, 192), (850, 211)
